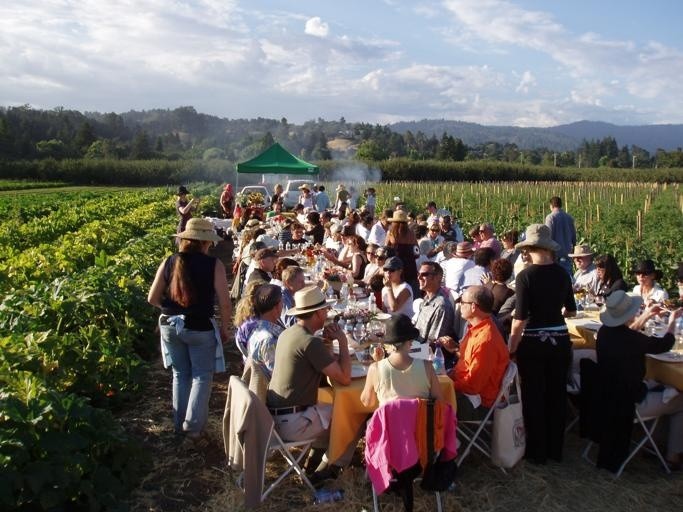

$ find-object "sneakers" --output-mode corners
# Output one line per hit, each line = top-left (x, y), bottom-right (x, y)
(302, 455), (342, 481)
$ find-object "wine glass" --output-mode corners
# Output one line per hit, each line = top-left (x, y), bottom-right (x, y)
(574, 282), (605, 317)
(223, 214), (226, 219)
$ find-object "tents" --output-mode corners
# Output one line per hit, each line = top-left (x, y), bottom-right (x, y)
(236, 142), (319, 193)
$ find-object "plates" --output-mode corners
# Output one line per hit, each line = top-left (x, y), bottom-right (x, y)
(314, 328), (324, 339)
(409, 340), (423, 349)
(351, 364), (368, 378)
(331, 346), (355, 357)
(372, 313), (394, 321)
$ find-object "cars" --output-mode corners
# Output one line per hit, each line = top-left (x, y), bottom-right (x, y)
(237, 185), (272, 210)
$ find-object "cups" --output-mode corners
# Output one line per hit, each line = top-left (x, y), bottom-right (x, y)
(343, 319), (388, 364)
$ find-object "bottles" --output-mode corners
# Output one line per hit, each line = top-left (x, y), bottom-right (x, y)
(433, 342), (445, 374)
(277, 240), (377, 319)
(311, 488), (345, 505)
(634, 306), (683, 361)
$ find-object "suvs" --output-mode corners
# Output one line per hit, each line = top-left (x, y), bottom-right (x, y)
(282, 178), (315, 211)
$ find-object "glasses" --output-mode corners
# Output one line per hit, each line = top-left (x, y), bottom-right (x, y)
(416, 270), (439, 278)
(458, 298), (479, 307)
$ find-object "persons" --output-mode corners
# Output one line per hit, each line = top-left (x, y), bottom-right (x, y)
(508, 197), (682, 473)
(176, 186), (200, 246)
(147, 217), (232, 451)
(220, 184), (529, 511)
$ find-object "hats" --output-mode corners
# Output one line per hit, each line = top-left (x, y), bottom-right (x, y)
(382, 314), (419, 343)
(170, 217), (225, 244)
(566, 242), (595, 258)
(239, 218), (267, 233)
(443, 239), (473, 258)
(222, 183), (234, 192)
(284, 285), (337, 317)
(386, 201), (437, 224)
(174, 185), (191, 196)
(337, 225), (355, 236)
(383, 256), (405, 271)
(252, 249), (280, 261)
(515, 224), (562, 253)
(599, 291), (643, 327)
(298, 180), (376, 194)
(627, 261), (662, 279)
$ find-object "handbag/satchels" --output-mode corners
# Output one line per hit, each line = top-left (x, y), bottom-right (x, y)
(421, 451), (457, 491)
(491, 393), (526, 466)
